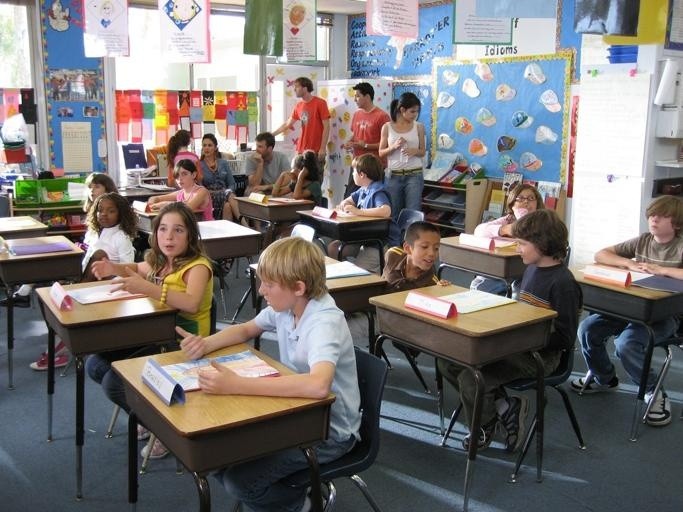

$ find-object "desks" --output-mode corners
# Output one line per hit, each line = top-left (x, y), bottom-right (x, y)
(1, 177), (680, 510)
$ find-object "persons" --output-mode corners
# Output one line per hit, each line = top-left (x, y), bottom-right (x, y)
(443, 209), (581, 454)
(148, 159), (214, 222)
(342, 82), (391, 201)
(469, 182), (545, 294)
(37, 171), (56, 180)
(29, 193), (139, 370)
(50, 71), (99, 119)
(245, 132), (291, 232)
(175, 237), (361, 511)
(346, 222), (452, 341)
(198, 134), (252, 231)
(271, 149), (322, 241)
(326, 154), (403, 260)
(271, 77), (330, 170)
(0, 172), (119, 307)
(168, 130), (202, 187)
(570, 196), (682, 426)
(379, 91), (425, 248)
(84, 199), (213, 461)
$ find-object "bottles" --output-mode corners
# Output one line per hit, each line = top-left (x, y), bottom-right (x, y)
(399, 136), (409, 164)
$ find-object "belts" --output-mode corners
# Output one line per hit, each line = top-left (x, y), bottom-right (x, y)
(391, 166), (423, 176)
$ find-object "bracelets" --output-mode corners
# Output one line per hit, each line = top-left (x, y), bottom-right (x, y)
(158, 284), (168, 308)
(273, 184), (281, 191)
(364, 143), (367, 150)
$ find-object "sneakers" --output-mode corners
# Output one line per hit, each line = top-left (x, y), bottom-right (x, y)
(140, 433), (172, 460)
(137, 424), (152, 441)
(462, 413), (499, 453)
(569, 374), (619, 394)
(28, 352), (70, 371)
(498, 393), (532, 455)
(0, 292), (31, 308)
(645, 385), (672, 426)
